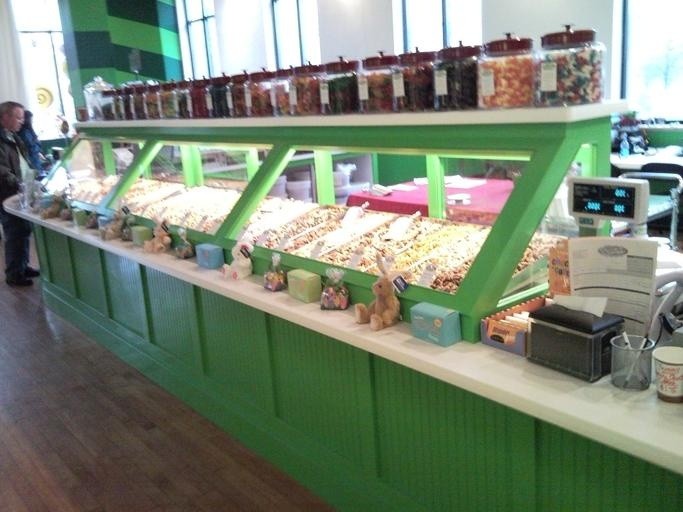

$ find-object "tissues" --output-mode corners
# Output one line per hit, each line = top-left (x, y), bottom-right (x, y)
(528, 293), (627, 383)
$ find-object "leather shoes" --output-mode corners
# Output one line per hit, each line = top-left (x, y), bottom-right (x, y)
(24, 266), (40, 277)
(5, 277), (33, 287)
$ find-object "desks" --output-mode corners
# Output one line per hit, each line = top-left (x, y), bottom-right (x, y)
(3, 192), (682, 510)
(611, 151), (682, 172)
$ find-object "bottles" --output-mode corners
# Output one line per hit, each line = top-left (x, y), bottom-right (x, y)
(133, 81), (148, 120)
(102, 90), (117, 120)
(189, 76), (210, 119)
(176, 78), (193, 120)
(145, 79), (162, 119)
(358, 51), (398, 113)
(230, 70), (251, 118)
(536, 25), (607, 107)
(208, 72), (233, 117)
(476, 31), (538, 110)
(323, 55), (359, 115)
(250, 67), (273, 117)
(269, 65), (292, 117)
(392, 47), (434, 113)
(160, 78), (180, 118)
(433, 42), (478, 112)
(292, 60), (320, 115)
(115, 82), (137, 121)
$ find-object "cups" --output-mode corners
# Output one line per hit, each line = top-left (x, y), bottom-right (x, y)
(653, 344), (683, 403)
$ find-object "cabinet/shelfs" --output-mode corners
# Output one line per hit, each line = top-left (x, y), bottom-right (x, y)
(612, 173), (683, 249)
(35, 98), (634, 344)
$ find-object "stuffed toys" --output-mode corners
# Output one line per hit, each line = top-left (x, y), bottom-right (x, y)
(222, 242), (254, 281)
(99, 209), (125, 240)
(145, 219), (171, 254)
(355, 277), (400, 330)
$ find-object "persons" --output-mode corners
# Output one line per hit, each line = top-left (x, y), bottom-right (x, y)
(0, 101), (40, 286)
(17, 110), (46, 182)
